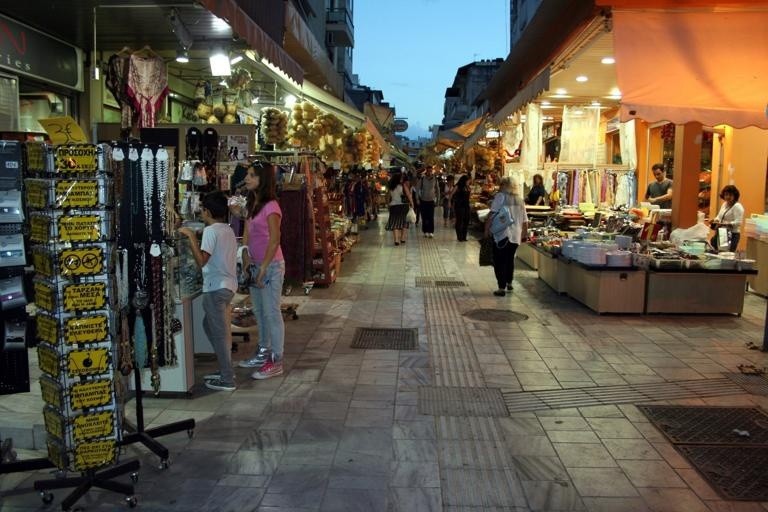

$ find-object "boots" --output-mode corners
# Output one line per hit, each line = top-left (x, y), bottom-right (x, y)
(238, 343), (284, 380)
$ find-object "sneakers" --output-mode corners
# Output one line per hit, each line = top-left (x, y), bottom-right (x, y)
(203, 370), (237, 392)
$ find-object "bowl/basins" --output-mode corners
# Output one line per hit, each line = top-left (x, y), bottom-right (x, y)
(678, 246), (706, 257)
(703, 250), (756, 270)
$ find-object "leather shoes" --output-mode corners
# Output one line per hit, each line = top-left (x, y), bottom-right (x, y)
(494, 285), (513, 296)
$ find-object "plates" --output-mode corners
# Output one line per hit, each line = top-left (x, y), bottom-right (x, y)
(558, 233), (637, 267)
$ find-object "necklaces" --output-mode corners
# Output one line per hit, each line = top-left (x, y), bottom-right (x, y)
(111, 149), (178, 396)
(188, 146), (216, 184)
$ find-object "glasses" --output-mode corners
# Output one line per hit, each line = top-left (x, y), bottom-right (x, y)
(252, 159), (264, 168)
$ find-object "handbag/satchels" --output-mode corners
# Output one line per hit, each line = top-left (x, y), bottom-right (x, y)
(490, 205), (514, 234)
(401, 195), (410, 203)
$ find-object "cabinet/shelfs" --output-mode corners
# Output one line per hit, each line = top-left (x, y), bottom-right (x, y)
(313, 181), (342, 288)
(515, 236), (768, 315)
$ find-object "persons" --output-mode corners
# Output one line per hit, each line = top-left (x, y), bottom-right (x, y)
(709, 185), (743, 252)
(241, 160), (286, 379)
(643, 163), (673, 209)
(484, 175), (528, 297)
(178, 191), (238, 392)
(385, 164), (472, 247)
(527, 174), (546, 219)
